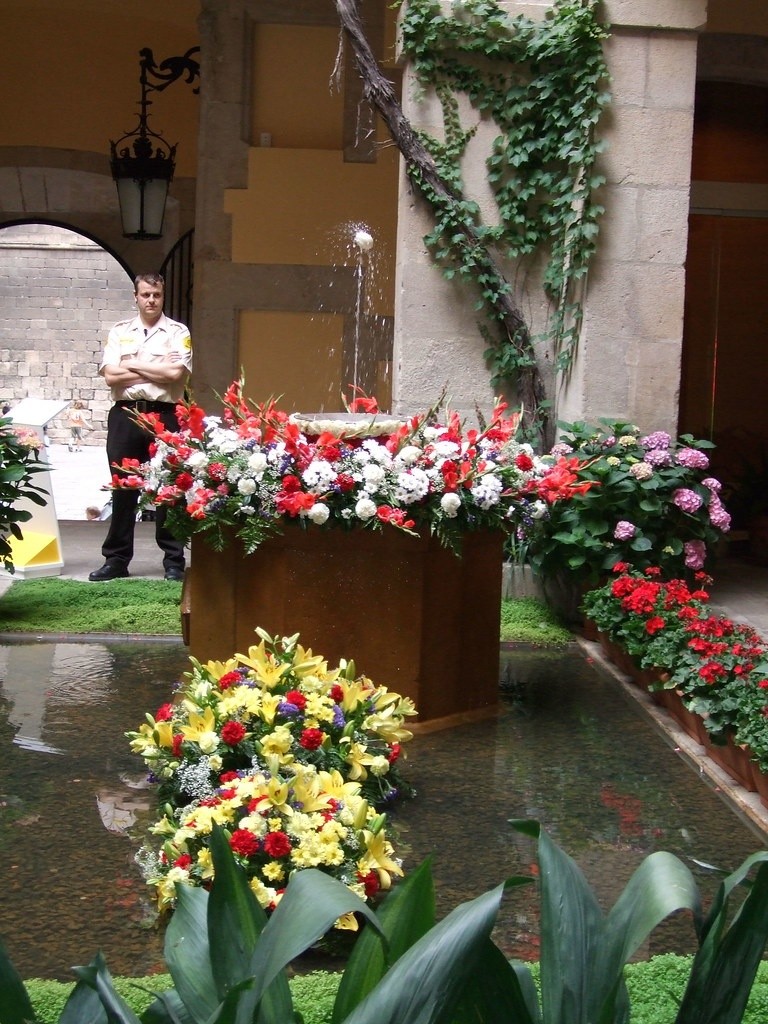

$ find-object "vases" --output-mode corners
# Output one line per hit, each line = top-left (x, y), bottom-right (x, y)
(582, 573), (768, 811)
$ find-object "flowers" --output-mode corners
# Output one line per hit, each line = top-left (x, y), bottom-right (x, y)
(0, 425), (41, 451)
(99, 362), (602, 560)
(580, 563), (768, 772)
(519, 418), (732, 575)
(125, 627), (418, 932)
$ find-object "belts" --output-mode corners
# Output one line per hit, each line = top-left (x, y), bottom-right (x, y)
(115, 400), (179, 413)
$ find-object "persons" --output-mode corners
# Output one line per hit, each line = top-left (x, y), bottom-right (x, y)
(89, 271), (194, 581)
(66, 401), (93, 452)
(44, 426), (49, 457)
(2, 406), (20, 436)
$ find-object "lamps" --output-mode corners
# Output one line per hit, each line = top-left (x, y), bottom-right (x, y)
(109, 47), (203, 242)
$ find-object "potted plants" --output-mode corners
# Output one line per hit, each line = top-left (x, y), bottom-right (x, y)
(727, 432), (768, 568)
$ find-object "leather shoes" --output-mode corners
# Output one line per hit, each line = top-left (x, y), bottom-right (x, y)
(164, 566), (182, 582)
(89, 565), (129, 581)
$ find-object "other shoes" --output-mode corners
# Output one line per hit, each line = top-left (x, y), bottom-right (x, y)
(76, 448), (82, 452)
(68, 446), (74, 452)
